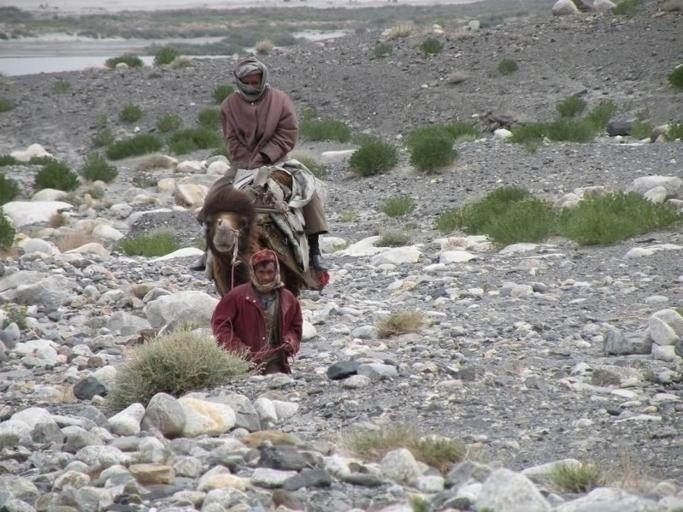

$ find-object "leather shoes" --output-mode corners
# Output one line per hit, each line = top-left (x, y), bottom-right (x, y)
(308, 252), (331, 273)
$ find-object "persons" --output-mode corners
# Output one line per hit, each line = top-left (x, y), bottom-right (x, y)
(187, 59), (330, 271)
(210, 249), (304, 376)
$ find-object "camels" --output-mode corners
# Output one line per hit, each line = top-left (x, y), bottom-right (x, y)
(199, 169), (301, 299)
